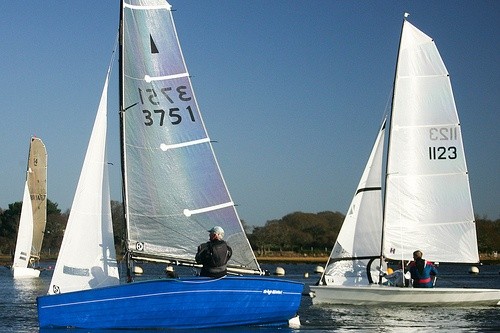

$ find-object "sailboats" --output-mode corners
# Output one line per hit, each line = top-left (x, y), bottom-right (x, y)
(36, 0), (306, 333)
(309, 12), (500, 307)
(10, 137), (48, 281)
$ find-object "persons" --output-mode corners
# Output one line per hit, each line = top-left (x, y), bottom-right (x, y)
(376, 263), (410, 290)
(195, 226), (233, 278)
(404, 251), (439, 288)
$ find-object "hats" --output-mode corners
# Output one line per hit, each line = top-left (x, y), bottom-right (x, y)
(208, 227), (224, 233)
